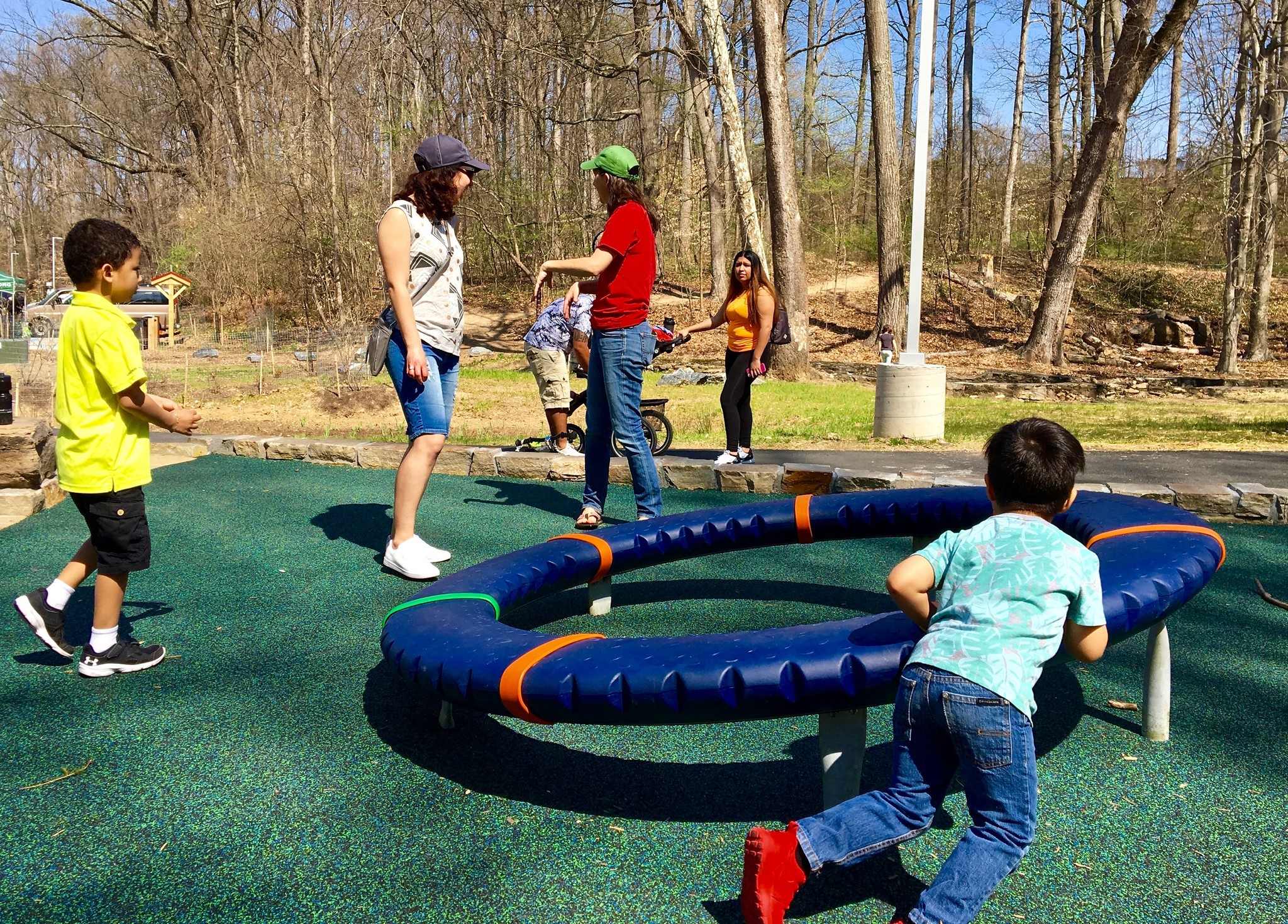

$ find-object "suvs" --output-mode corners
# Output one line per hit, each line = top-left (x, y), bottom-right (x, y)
(22, 283), (182, 338)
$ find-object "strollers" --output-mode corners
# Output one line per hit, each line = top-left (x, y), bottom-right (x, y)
(515, 313), (692, 458)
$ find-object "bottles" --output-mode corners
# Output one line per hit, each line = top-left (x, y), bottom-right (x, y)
(0, 372), (13, 425)
(663, 317), (675, 333)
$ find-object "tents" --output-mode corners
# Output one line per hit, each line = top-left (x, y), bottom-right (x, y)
(0, 272), (27, 323)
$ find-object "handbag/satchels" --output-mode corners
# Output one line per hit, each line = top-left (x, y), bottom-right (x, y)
(750, 284), (792, 345)
(368, 315), (396, 376)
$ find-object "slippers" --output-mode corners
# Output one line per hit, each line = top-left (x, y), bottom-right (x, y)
(576, 512), (603, 526)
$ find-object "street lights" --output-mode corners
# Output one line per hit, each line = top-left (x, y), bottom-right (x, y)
(11, 252), (19, 277)
(52, 236), (63, 290)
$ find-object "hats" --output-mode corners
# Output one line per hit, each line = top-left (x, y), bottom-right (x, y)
(580, 145), (641, 180)
(414, 135), (491, 174)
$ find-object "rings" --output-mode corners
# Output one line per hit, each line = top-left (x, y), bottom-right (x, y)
(409, 367), (415, 370)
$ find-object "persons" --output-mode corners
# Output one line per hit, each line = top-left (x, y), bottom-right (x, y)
(738, 417), (1110, 924)
(377, 135), (491, 580)
(878, 324), (898, 364)
(524, 292), (606, 457)
(15, 218), (203, 677)
(675, 250), (782, 463)
(531, 145), (661, 530)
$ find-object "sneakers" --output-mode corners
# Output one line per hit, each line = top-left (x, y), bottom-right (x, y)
(743, 822), (807, 924)
(79, 636), (166, 677)
(714, 450), (742, 464)
(14, 587), (74, 656)
(738, 447), (755, 464)
(387, 533), (451, 563)
(384, 537), (440, 579)
(556, 442), (586, 456)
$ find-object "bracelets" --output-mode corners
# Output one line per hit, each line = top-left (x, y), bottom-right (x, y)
(169, 411), (179, 433)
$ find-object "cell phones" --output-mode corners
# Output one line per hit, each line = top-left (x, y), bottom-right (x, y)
(746, 363), (766, 377)
